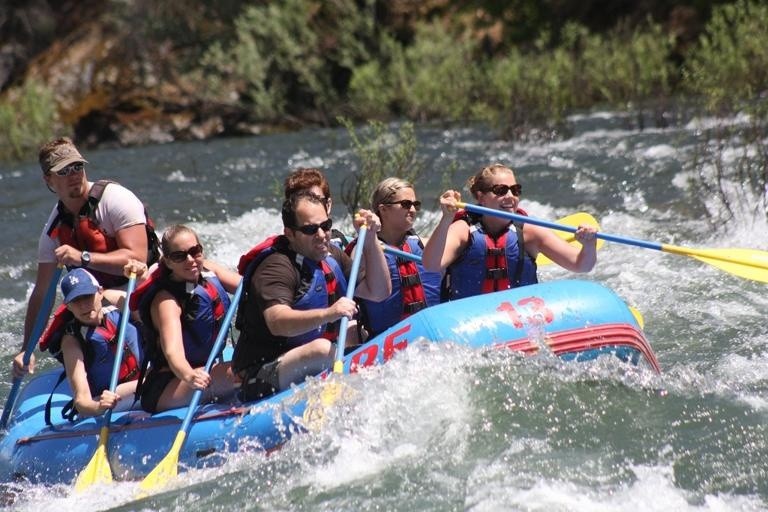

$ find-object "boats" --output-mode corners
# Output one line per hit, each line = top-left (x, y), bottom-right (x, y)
(1, 279), (659, 491)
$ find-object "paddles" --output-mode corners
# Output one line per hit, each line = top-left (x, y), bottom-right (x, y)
(536, 212), (605, 265)
(73, 266), (137, 494)
(134, 277), (243, 502)
(305, 213), (371, 432)
(447, 199), (768, 283)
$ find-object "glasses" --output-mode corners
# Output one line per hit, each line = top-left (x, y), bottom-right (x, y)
(170, 247), (204, 263)
(54, 163), (84, 177)
(380, 200), (421, 211)
(477, 184), (522, 197)
(289, 220), (332, 236)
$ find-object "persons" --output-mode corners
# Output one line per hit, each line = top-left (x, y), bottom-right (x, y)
(12, 137), (163, 380)
(60, 260), (147, 417)
(230, 164), (596, 402)
(130, 225), (246, 413)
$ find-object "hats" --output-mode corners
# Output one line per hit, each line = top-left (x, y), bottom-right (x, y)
(40, 142), (88, 173)
(60, 268), (101, 304)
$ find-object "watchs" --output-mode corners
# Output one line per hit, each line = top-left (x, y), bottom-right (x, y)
(81, 251), (91, 267)
